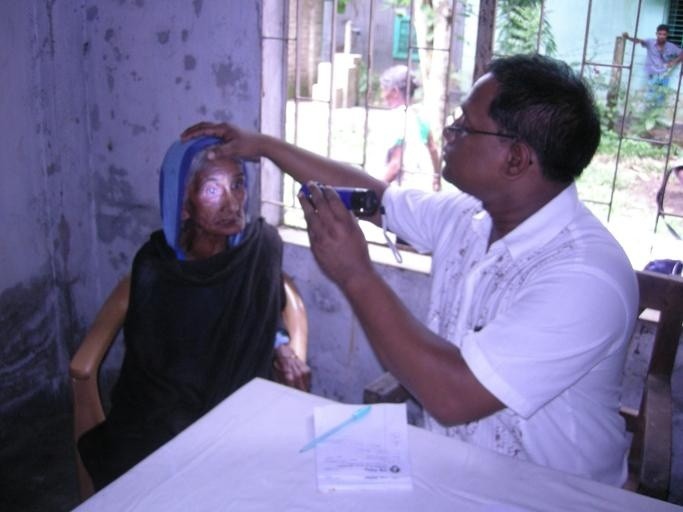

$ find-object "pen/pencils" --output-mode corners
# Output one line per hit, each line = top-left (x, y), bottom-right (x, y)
(297, 403), (372, 455)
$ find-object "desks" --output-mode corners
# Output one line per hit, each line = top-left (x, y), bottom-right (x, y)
(67, 376), (682, 512)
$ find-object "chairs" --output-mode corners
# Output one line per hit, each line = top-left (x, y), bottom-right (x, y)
(69, 268), (309, 500)
(618, 268), (683, 502)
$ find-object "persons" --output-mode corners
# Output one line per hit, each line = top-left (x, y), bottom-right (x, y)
(370, 64), (442, 191)
(178, 54), (641, 490)
(77, 136), (313, 494)
(621, 24), (683, 113)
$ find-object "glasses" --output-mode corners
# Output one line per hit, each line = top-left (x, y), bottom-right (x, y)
(443, 106), (515, 139)
